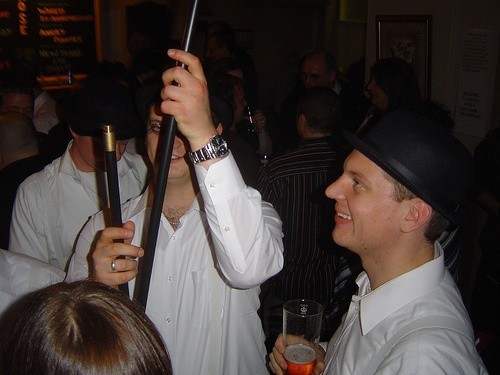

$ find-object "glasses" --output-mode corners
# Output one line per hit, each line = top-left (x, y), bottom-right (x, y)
(302, 69), (333, 82)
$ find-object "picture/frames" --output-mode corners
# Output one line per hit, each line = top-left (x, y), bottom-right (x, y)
(374, 14), (431, 105)
(331, 19), (365, 73)
(231, 27), (255, 50)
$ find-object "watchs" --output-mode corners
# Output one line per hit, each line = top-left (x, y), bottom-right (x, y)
(188, 134), (228, 165)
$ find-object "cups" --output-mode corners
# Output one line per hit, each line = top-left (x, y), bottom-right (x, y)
(247, 104), (259, 124)
(280, 299), (323, 374)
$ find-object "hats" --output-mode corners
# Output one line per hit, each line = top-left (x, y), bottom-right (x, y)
(66, 72), (141, 140)
(340, 100), (465, 227)
(138, 52), (235, 131)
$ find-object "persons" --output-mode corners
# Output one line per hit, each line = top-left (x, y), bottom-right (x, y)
(267, 119), (489, 375)
(278, 50), (373, 153)
(64, 76), (285, 375)
(255, 86), (359, 375)
(0, 248), (66, 317)
(8, 75), (151, 276)
(352, 57), (421, 138)
(3, 12), (275, 156)
(470, 128), (500, 375)
(1, 280), (174, 375)
(0, 111), (51, 252)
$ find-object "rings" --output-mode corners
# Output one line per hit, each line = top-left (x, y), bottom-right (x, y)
(111, 261), (115, 270)
